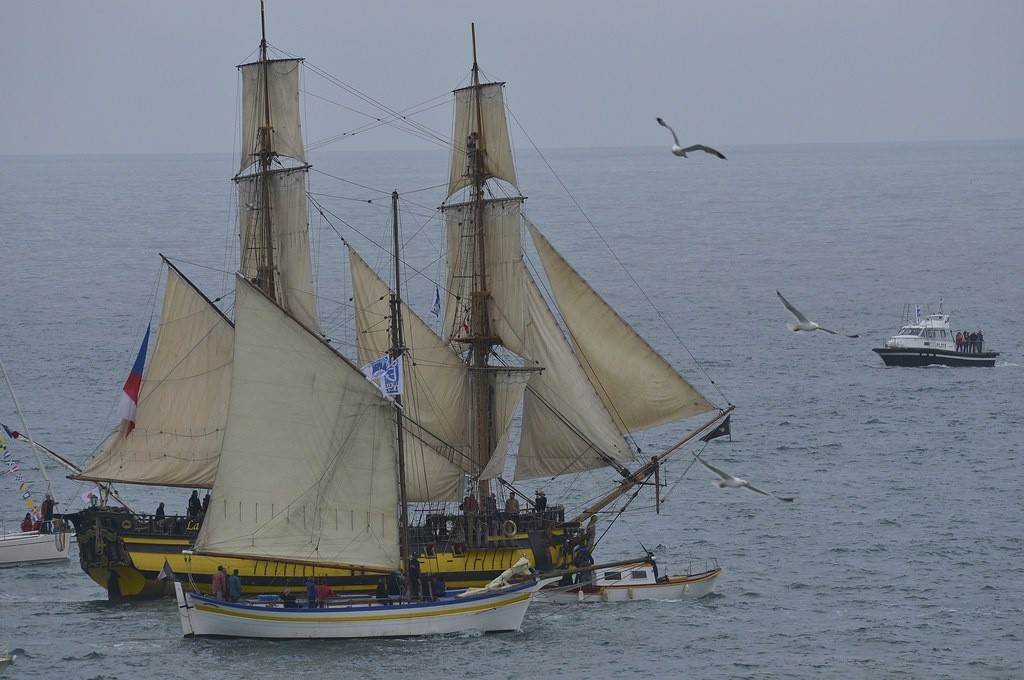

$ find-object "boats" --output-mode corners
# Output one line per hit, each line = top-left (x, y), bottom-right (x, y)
(872, 299), (1000, 370)
(0, 362), (74, 563)
(540, 549), (722, 602)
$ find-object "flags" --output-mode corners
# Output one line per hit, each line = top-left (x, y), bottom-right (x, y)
(81, 486), (101, 504)
(117, 325), (150, 440)
(699, 415), (730, 443)
(154, 559), (172, 583)
(0, 436), (39, 514)
(360, 355), (404, 398)
(430, 285), (441, 318)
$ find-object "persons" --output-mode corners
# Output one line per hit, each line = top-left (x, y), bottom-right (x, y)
(375, 569), (405, 605)
(188, 490), (210, 536)
(410, 551), (421, 598)
(32, 518), (42, 531)
(306, 576), (332, 608)
(212, 566), (242, 603)
(505, 491), (519, 515)
(459, 494), (478, 515)
(955, 330), (983, 353)
(155, 502), (165, 520)
(21, 512), (32, 532)
(42, 494), (54, 533)
(490, 492), (497, 512)
(534, 491), (547, 512)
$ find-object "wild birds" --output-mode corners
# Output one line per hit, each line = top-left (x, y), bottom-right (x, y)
(656, 116), (728, 162)
(774, 288), (860, 339)
(691, 451), (795, 503)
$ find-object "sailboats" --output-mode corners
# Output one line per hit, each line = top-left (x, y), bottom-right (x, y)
(172, 191), (561, 638)
(52, 0), (740, 598)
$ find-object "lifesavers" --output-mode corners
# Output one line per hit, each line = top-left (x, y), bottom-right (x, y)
(503, 520), (517, 537)
